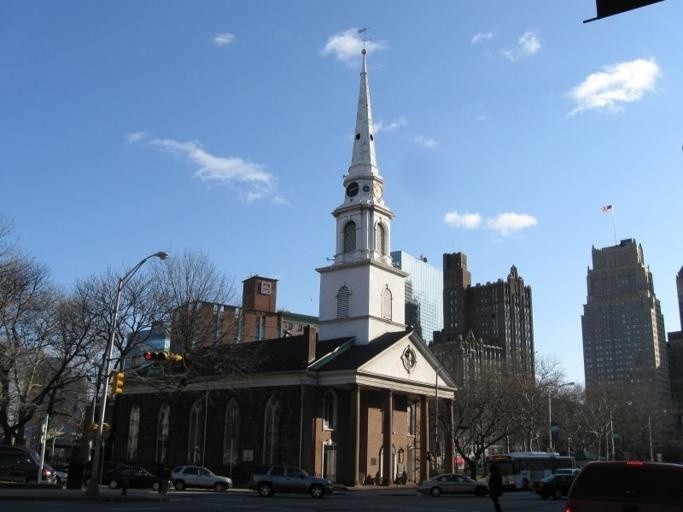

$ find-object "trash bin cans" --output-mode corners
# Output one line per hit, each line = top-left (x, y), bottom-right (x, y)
(65, 460), (83, 489)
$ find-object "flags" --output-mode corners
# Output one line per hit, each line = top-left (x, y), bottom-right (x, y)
(600, 203), (613, 214)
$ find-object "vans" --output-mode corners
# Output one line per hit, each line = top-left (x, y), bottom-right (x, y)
(0, 446), (56, 488)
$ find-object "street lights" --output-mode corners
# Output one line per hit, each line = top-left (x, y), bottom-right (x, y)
(648, 410), (667, 462)
(434, 364), (454, 476)
(86, 251), (168, 496)
(611, 401), (633, 457)
(548, 381), (576, 452)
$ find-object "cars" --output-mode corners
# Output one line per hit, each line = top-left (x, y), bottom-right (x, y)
(101, 465), (232, 492)
(248, 463), (332, 498)
(528, 460), (683, 511)
(414, 474), (488, 498)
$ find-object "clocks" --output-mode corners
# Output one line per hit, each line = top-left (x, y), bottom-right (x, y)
(373, 183), (383, 198)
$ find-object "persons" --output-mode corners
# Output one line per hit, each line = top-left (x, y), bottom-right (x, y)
(157, 464), (171, 494)
(487, 463), (504, 512)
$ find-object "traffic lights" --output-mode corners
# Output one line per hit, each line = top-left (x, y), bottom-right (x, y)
(117, 372), (125, 395)
(144, 351), (168, 361)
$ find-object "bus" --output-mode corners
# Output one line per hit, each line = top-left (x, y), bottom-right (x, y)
(486, 452), (576, 492)
